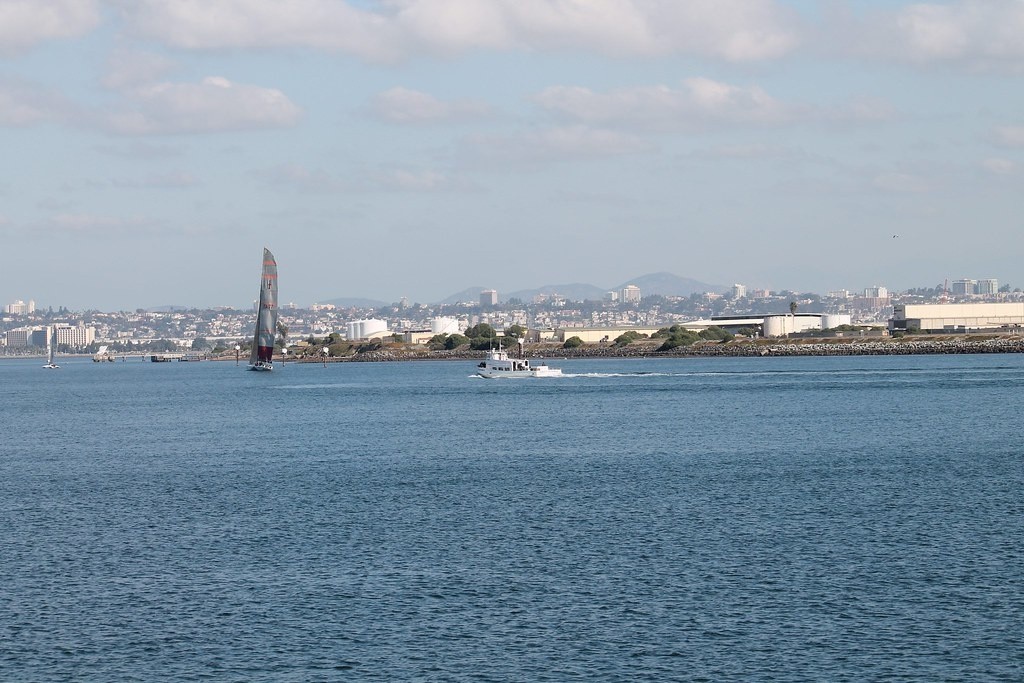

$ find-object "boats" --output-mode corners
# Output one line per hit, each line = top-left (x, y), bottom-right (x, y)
(530, 365), (563, 377)
(475, 339), (534, 378)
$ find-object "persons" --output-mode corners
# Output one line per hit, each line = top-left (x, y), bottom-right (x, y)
(542, 362), (545, 366)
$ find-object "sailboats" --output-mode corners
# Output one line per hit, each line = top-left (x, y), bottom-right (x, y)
(41, 336), (60, 369)
(246, 247), (279, 371)
